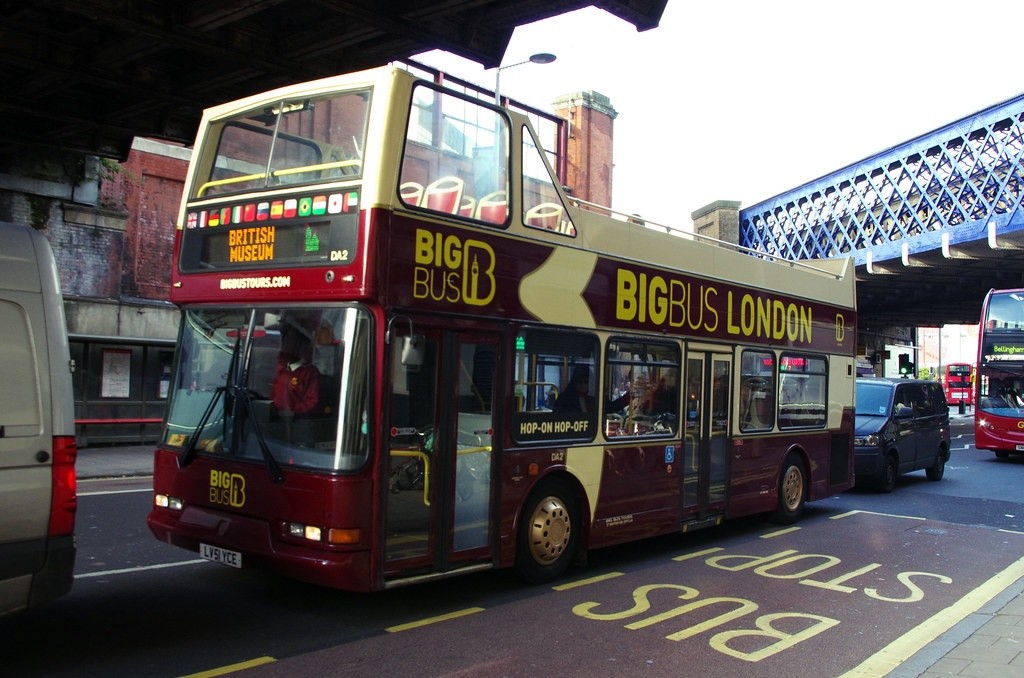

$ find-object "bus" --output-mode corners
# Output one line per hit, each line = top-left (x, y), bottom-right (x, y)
(147, 57), (858, 597)
(974, 288), (1024, 460)
(945, 363), (977, 404)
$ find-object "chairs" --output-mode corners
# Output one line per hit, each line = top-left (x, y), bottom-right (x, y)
(304, 375), (337, 418)
(688, 375), (805, 431)
(399, 175), (575, 236)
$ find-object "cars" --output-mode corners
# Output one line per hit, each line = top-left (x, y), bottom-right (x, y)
(852, 377), (951, 491)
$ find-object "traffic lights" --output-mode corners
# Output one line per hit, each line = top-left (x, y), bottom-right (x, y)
(899, 354), (909, 374)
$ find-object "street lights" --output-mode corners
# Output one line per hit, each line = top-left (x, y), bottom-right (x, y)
(494, 53), (556, 217)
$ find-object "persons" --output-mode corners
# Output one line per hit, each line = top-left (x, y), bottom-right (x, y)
(272, 341), (325, 418)
(553, 368), (592, 414)
(639, 376), (678, 417)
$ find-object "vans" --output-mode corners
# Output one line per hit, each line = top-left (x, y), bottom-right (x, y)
(2, 223), (77, 616)
